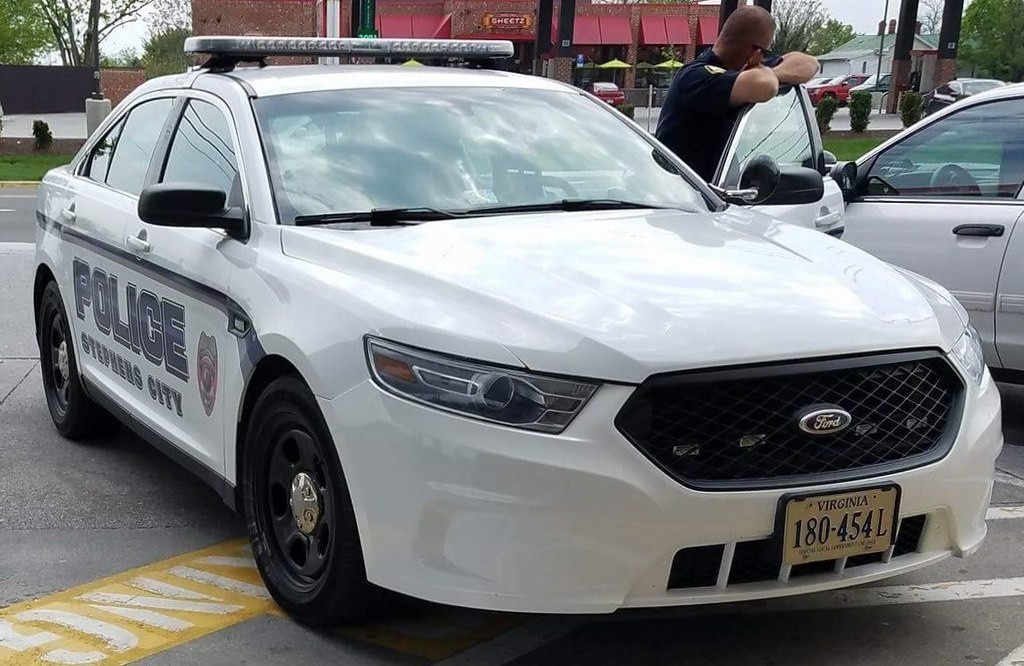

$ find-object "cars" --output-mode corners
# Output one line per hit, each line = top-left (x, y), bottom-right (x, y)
(777, 81), (1024, 388)
(800, 77), (849, 94)
(914, 78), (1007, 125)
(585, 81), (626, 107)
(27, 32), (1006, 634)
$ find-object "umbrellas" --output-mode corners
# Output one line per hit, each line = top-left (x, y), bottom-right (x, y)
(637, 62), (653, 68)
(599, 58), (632, 83)
(583, 63), (598, 69)
(655, 59), (683, 68)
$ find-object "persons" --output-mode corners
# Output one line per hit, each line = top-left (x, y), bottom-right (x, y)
(655, 4), (820, 182)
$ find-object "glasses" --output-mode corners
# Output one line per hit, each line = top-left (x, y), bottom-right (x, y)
(753, 44), (774, 59)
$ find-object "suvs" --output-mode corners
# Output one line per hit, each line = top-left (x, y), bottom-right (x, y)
(805, 73), (873, 109)
(846, 72), (891, 109)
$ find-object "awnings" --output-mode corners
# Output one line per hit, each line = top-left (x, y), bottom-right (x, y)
(380, 13), (451, 39)
(572, 16), (632, 45)
(639, 15), (691, 45)
(697, 16), (718, 45)
